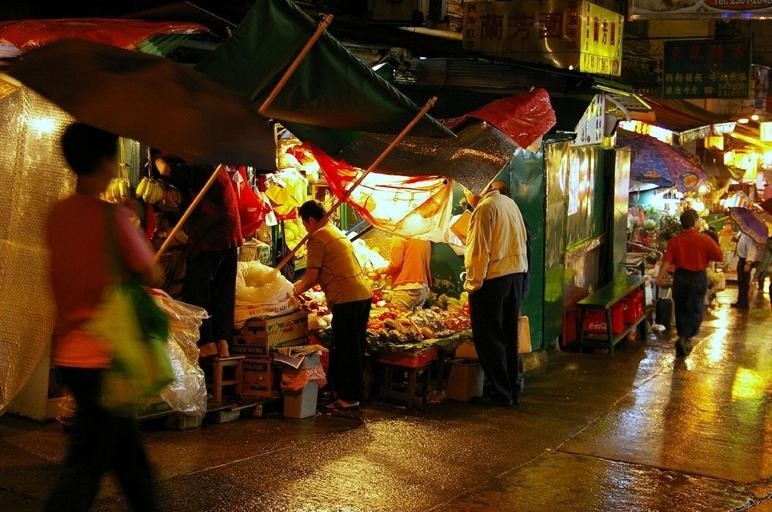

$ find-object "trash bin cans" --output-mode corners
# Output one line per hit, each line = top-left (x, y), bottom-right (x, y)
(443, 358), (486, 402)
(283, 355), (320, 419)
(656, 298), (672, 331)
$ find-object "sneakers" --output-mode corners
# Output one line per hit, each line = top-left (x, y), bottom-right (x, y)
(320, 399), (360, 409)
(675, 339), (692, 356)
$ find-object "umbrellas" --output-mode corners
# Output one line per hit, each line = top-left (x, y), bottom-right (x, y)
(612, 126), (709, 192)
(728, 190), (772, 246)
(2, 36), (277, 169)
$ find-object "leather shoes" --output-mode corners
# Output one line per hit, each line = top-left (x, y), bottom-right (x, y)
(731, 302), (747, 308)
(469, 395), (519, 406)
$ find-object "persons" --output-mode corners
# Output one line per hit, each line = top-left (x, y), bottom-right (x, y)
(37, 118), (178, 509)
(164, 156), (239, 370)
(460, 185), (527, 410)
(375, 235), (433, 313)
(655, 209), (725, 357)
(287, 199), (372, 415)
(643, 225), (725, 332)
(730, 229), (772, 310)
(490, 180), (533, 398)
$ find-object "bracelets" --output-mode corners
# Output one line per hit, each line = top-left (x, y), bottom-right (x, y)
(293, 283), (298, 295)
(381, 267), (386, 274)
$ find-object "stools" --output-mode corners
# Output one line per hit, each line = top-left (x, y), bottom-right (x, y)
(207, 355), (248, 408)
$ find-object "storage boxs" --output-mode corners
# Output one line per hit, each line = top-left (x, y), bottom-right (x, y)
(232, 311), (308, 356)
(239, 356), (283, 400)
(583, 300), (626, 333)
(619, 288), (644, 324)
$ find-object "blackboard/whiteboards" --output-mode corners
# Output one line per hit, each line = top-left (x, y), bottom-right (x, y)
(662, 37), (752, 99)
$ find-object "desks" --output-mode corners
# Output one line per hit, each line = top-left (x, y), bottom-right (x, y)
(378, 328), (479, 412)
(575, 273), (649, 359)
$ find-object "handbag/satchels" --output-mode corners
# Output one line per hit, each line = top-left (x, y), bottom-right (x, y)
(94, 203), (176, 419)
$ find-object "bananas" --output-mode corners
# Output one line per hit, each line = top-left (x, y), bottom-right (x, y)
(136, 175), (164, 204)
(100, 177), (129, 205)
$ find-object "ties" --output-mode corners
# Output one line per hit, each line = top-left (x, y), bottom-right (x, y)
(734, 234), (742, 256)
